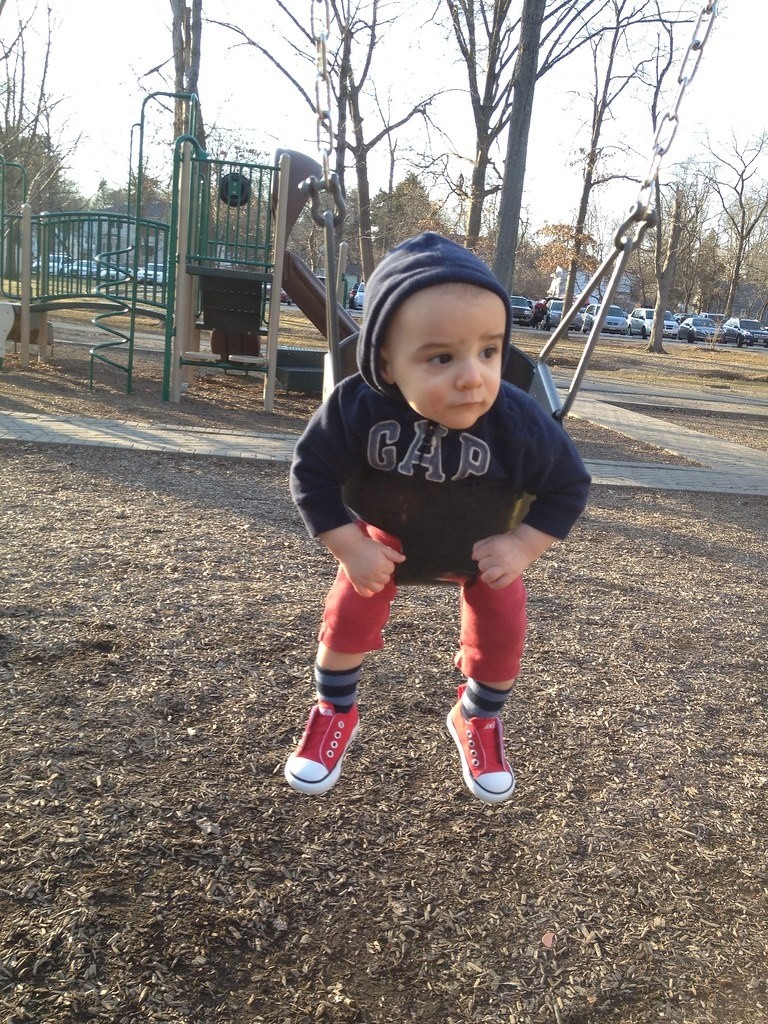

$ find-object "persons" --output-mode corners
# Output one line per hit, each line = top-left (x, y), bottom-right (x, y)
(532, 298), (547, 328)
(282, 232), (593, 804)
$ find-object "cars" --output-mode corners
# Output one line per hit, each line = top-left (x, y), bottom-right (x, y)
(349, 282), (365, 309)
(678, 318), (725, 343)
(722, 317), (768, 348)
(673, 313), (696, 324)
(699, 313), (726, 326)
(32, 254), (75, 275)
(262, 281), (294, 305)
(99, 263), (132, 281)
(510, 296), (535, 324)
(532, 297), (582, 331)
(627, 309), (679, 340)
(69, 260), (97, 278)
(136, 262), (169, 285)
(581, 304), (628, 335)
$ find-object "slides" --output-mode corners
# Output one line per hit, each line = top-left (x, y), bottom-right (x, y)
(282, 249), (360, 344)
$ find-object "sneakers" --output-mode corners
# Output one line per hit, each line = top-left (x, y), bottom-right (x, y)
(447, 681), (516, 802)
(284, 688), (358, 796)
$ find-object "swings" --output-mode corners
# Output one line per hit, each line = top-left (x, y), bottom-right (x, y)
(313, 84), (690, 587)
